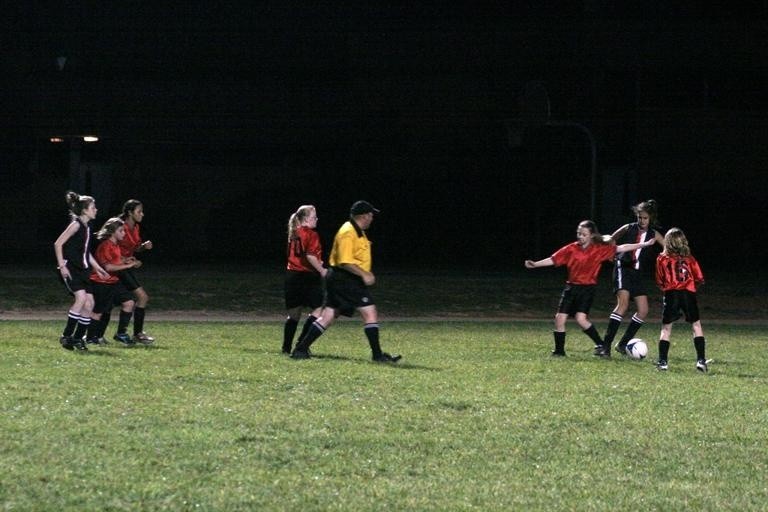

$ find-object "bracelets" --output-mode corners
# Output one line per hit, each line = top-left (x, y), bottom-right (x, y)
(57, 260), (68, 269)
(315, 264), (324, 273)
(640, 242), (648, 248)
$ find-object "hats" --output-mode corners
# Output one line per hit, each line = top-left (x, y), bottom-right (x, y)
(349, 200), (381, 215)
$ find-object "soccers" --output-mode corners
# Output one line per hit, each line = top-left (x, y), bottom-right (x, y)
(626, 338), (648, 360)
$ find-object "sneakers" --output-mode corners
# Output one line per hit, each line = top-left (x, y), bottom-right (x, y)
(289, 346), (319, 360)
(590, 342), (628, 358)
(652, 359), (669, 371)
(58, 330), (155, 350)
(372, 353), (402, 362)
(695, 357), (710, 374)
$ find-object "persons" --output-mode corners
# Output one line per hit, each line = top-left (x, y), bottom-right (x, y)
(602, 199), (667, 360)
(54, 190), (110, 353)
(98, 199), (155, 344)
(653, 227), (711, 372)
(283, 204), (330, 354)
(89, 217), (138, 347)
(292, 200), (402, 362)
(525, 219), (656, 357)
